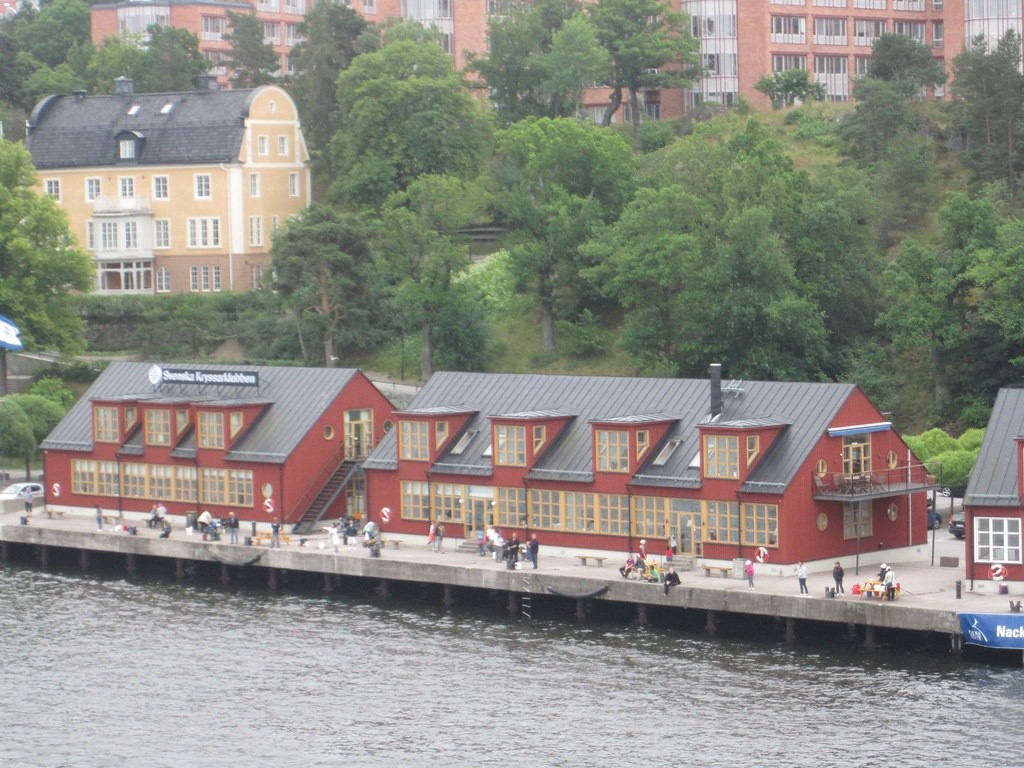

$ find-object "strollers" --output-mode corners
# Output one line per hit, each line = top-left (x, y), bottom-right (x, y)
(202, 520), (220, 542)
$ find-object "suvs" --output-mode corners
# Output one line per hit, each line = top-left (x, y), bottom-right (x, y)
(948, 509), (966, 538)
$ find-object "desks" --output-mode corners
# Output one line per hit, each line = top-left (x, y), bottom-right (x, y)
(844, 476), (871, 481)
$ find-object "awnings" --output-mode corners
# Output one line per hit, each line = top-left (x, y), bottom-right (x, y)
(826, 422), (892, 437)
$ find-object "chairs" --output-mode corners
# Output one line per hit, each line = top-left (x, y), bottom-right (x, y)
(813, 476), (830, 496)
(838, 474), (885, 496)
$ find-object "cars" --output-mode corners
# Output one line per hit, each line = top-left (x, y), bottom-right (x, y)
(927, 507), (943, 531)
(0, 482), (43, 499)
(937, 482), (966, 497)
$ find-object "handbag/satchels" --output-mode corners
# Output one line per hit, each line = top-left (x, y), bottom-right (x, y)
(885, 580), (893, 586)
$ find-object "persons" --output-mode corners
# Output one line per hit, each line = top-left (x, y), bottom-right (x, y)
(24, 486), (33, 518)
(832, 562), (844, 596)
(186, 511), (239, 544)
(478, 524), (538, 570)
(93, 503), (103, 532)
(157, 518), (171, 538)
(429, 519), (442, 552)
(669, 532), (677, 554)
(324, 509), (380, 557)
(795, 560), (808, 596)
(620, 554), (658, 583)
(639, 540), (647, 560)
(744, 560), (755, 590)
(877, 563), (888, 582)
(882, 566), (897, 601)
(665, 545), (673, 561)
(149, 502), (168, 528)
(270, 517), (281, 548)
(661, 566), (682, 597)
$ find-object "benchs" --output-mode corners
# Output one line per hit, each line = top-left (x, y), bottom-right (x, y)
(701, 565), (733, 579)
(381, 537), (402, 549)
(45, 510), (66, 520)
(858, 580), (898, 601)
(102, 513), (120, 524)
(575, 554), (608, 568)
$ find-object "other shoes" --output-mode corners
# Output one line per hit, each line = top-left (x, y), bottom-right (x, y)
(841, 593), (845, 596)
(835, 593), (839, 596)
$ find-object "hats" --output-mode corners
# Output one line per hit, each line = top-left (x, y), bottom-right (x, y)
(880, 564), (887, 569)
(744, 560), (751, 565)
(885, 566), (891, 571)
(640, 540), (647, 545)
